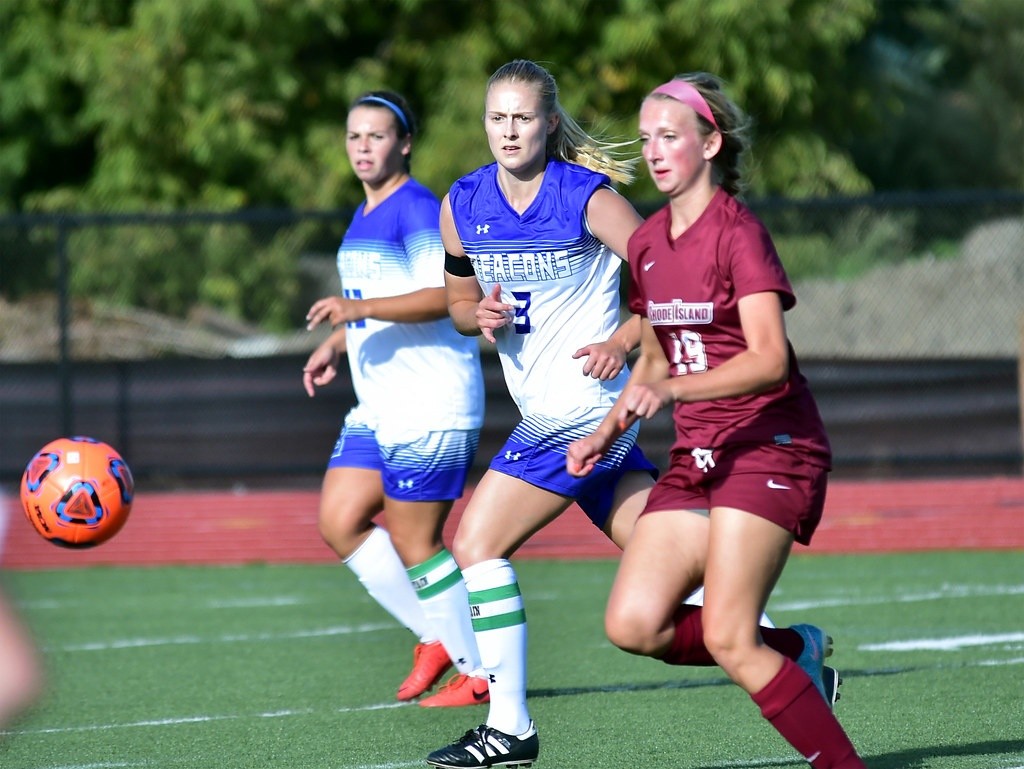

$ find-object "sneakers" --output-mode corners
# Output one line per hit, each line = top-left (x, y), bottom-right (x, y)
(396, 637), (449, 701)
(424, 721), (540, 768)
(418, 674), (490, 708)
(789, 623), (832, 707)
(820, 663), (842, 712)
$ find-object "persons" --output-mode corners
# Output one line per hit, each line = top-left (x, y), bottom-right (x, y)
(425, 60), (841, 769)
(567, 72), (867, 768)
(302, 92), (490, 706)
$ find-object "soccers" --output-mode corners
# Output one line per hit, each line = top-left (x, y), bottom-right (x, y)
(20, 435), (135, 548)
